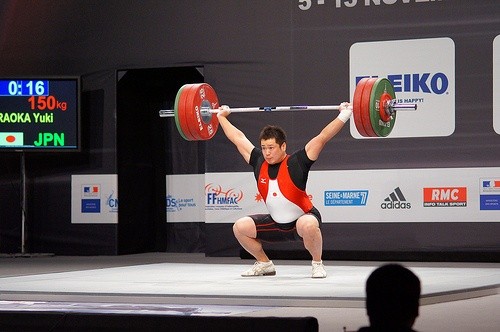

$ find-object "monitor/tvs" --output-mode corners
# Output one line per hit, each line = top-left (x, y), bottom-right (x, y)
(0, 75), (83, 154)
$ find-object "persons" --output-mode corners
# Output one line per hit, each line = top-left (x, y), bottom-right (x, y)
(217, 102), (352, 278)
(354, 263), (422, 332)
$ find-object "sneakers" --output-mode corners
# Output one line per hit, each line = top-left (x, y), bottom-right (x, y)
(242, 261), (276, 277)
(312, 259), (326, 278)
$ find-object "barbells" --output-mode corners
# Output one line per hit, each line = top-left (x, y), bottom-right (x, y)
(158, 77), (417, 138)
